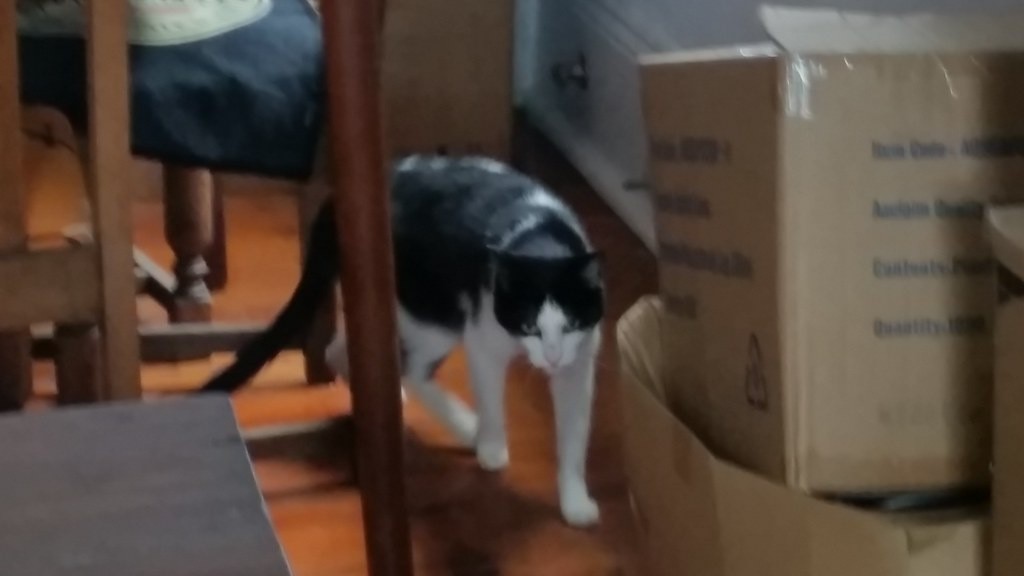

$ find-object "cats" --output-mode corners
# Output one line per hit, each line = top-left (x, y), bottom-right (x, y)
(190, 153), (606, 527)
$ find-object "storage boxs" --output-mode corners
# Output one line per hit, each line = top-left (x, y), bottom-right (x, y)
(380, 0), (515, 165)
(614, 294), (989, 576)
(634, 6), (1024, 495)
